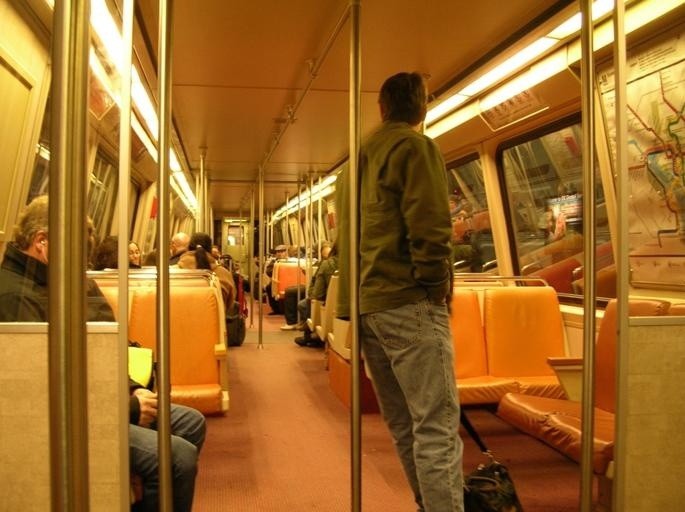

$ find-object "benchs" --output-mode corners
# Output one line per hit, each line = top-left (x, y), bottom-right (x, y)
(82, 265), (231, 416)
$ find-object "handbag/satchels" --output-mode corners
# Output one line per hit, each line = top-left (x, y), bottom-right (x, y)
(464, 448), (522, 511)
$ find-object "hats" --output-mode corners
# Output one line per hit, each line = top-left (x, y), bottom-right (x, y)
(269, 245), (287, 254)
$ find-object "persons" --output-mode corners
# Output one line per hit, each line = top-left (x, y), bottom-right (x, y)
(447, 188), (474, 222)
(545, 208), (567, 243)
(92, 231), (338, 350)
(332, 69), (466, 511)
(0, 195), (207, 512)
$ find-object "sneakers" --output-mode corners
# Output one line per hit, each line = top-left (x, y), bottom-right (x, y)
(281, 323), (297, 331)
(295, 337), (322, 347)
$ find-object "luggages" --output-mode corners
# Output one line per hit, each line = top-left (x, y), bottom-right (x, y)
(219, 256), (246, 346)
